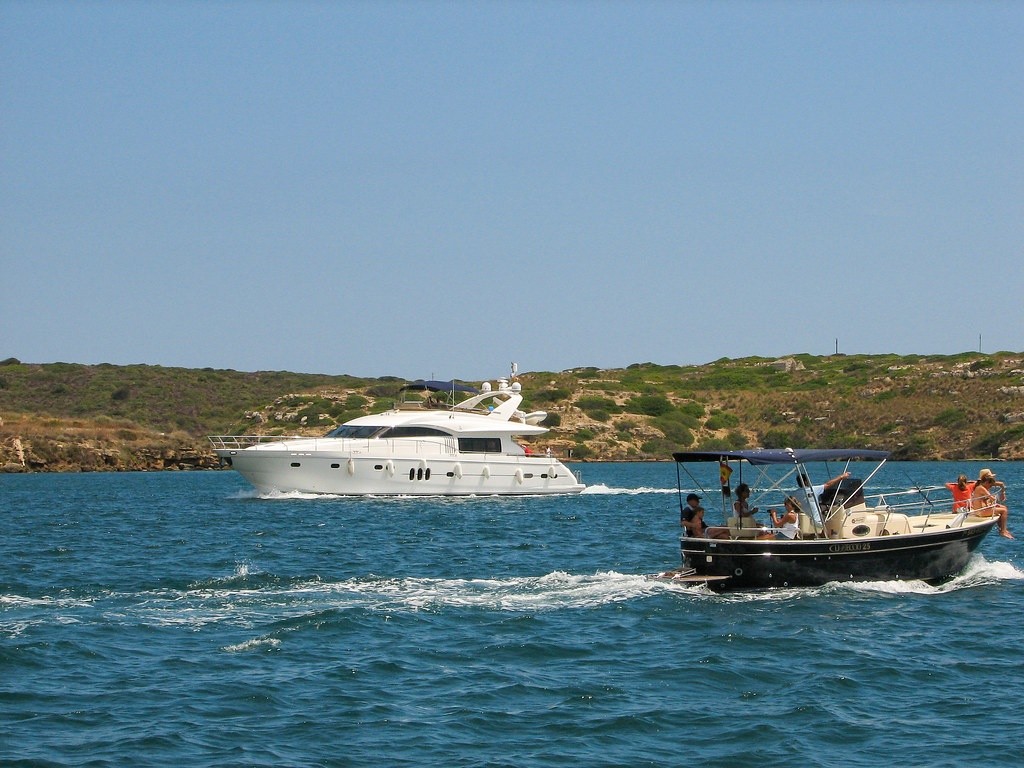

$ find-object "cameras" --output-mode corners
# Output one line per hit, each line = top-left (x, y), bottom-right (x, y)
(767, 509), (771, 513)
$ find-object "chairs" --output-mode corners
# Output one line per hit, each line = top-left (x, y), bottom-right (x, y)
(729, 516), (762, 540)
(799, 514), (822, 537)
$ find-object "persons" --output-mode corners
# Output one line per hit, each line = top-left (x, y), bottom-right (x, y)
(973, 469), (1014, 539)
(794, 472), (852, 528)
(732, 483), (759, 517)
(753, 495), (801, 540)
(681, 493), (731, 539)
(944, 475), (977, 514)
(525, 444), (534, 457)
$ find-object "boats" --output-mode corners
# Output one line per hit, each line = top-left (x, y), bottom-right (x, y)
(206, 361), (587, 499)
(669, 444), (1005, 597)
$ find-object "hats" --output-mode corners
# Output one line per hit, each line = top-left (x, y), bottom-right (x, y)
(686, 493), (703, 502)
(977, 469), (996, 480)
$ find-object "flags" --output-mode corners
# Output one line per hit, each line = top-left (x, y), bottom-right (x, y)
(720, 462), (733, 484)
